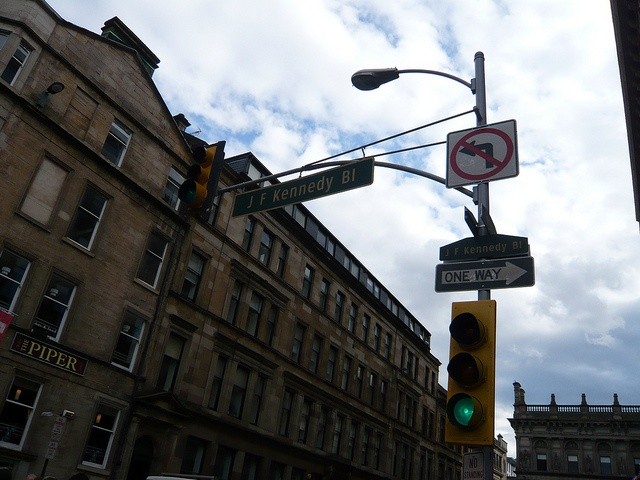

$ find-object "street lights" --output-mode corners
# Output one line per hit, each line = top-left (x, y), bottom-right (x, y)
(350, 51), (498, 478)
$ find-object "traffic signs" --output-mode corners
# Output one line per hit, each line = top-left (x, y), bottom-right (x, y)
(231, 156), (375, 216)
(434, 254), (535, 293)
(439, 232), (530, 260)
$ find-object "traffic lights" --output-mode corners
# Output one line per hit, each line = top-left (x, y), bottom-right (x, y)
(178, 141), (227, 211)
(446, 301), (498, 442)
